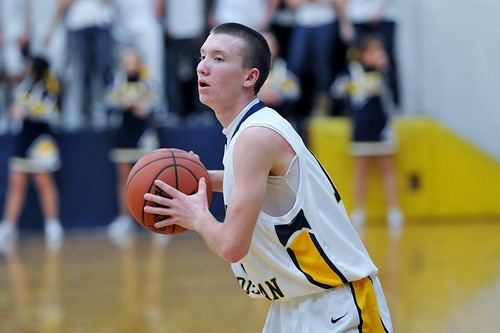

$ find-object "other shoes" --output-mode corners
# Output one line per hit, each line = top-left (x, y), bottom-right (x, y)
(45, 218), (63, 239)
(388, 211), (403, 227)
(0, 221), (15, 241)
(328, 99), (346, 115)
(351, 209), (365, 225)
(109, 217), (134, 236)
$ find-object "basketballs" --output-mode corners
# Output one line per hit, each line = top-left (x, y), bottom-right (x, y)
(124, 148), (212, 235)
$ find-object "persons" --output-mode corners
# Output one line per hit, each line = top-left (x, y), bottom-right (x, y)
(143, 22), (393, 333)
(0, 0), (403, 242)
